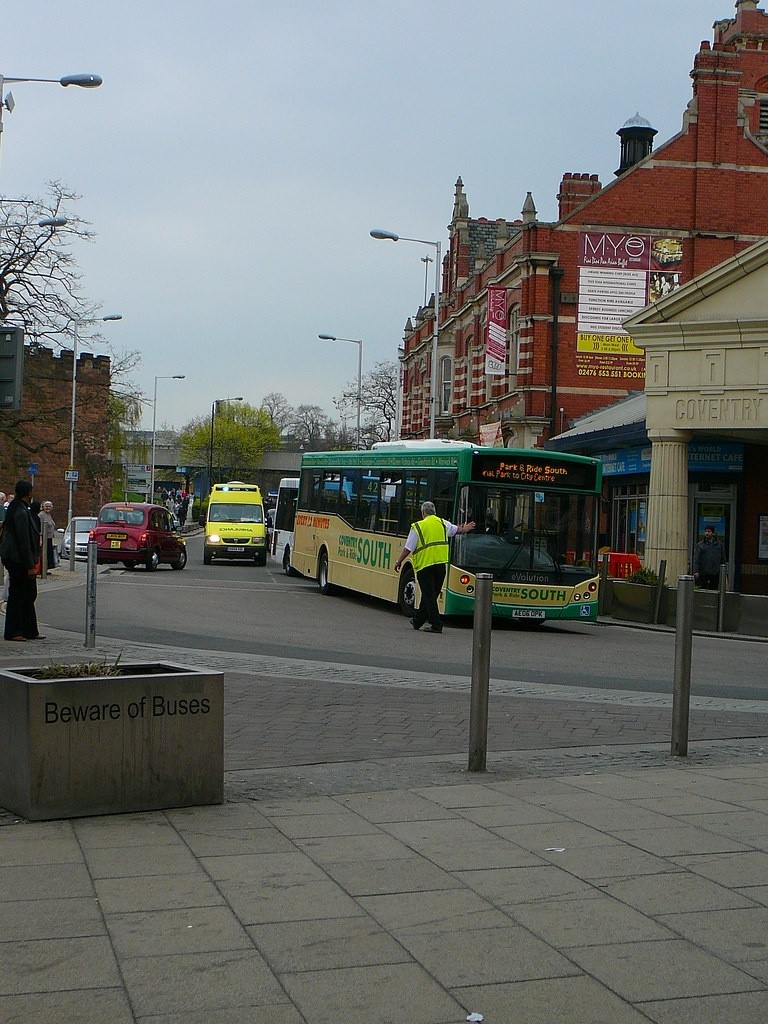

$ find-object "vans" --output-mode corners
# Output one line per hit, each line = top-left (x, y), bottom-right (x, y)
(199, 483), (271, 566)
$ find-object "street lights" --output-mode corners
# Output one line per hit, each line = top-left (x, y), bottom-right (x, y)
(210, 396), (244, 488)
(67, 315), (120, 524)
(320, 334), (362, 450)
(371, 228), (442, 441)
(151, 375), (186, 501)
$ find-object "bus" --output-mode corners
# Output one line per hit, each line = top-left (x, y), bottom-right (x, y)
(292, 441), (605, 624)
(270, 477), (428, 577)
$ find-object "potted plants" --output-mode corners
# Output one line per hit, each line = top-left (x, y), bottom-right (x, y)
(668, 581), (740, 632)
(611, 565), (668, 624)
(0, 657), (224, 821)
(598, 562), (623, 616)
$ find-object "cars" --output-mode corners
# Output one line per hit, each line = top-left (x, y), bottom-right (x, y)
(264, 493), (277, 526)
(59, 517), (99, 563)
(90, 502), (189, 570)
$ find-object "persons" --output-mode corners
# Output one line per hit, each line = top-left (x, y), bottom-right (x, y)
(0, 491), (56, 616)
(0, 479), (47, 641)
(692, 526), (729, 591)
(485, 513), (498, 535)
(394, 501), (475, 633)
(156, 486), (195, 527)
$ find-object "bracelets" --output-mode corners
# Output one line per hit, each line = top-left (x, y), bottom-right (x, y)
(396, 561), (400, 565)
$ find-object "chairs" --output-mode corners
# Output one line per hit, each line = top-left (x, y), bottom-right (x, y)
(320, 498), (420, 535)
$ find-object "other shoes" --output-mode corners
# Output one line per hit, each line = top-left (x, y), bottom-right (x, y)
(8, 634), (46, 642)
(410, 619), (442, 633)
